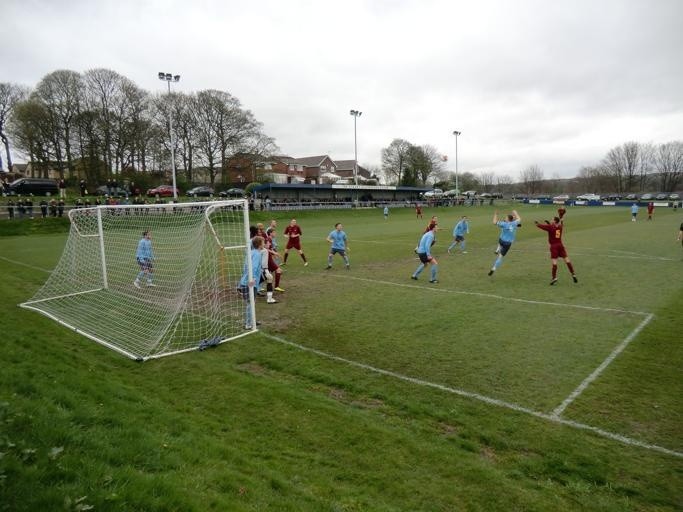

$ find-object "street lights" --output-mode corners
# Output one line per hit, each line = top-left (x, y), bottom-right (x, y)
(157, 71), (180, 199)
(350, 110), (362, 185)
(452, 131), (460, 198)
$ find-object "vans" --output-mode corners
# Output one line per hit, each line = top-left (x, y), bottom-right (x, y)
(1, 177), (58, 197)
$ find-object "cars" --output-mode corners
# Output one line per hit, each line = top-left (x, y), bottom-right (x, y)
(185, 185), (216, 197)
(424, 188), (503, 199)
(514, 193), (681, 201)
(146, 185), (180, 197)
(218, 188), (244, 197)
(95, 185), (127, 196)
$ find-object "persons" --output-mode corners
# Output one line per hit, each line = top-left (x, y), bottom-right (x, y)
(488, 209), (521, 276)
(237, 216), (309, 329)
(647, 202), (654, 221)
(414, 216), (439, 254)
(133, 231), (156, 290)
(324, 223), (350, 270)
(631, 203), (638, 221)
(367, 191), (480, 220)
(676, 222), (683, 247)
(558, 207), (565, 225)
(2, 179), (345, 219)
(411, 224), (440, 284)
(535, 216), (577, 285)
(447, 216), (470, 255)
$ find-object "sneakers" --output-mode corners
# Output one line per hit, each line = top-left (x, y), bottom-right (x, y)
(256, 320), (261, 325)
(551, 277), (558, 284)
(325, 265), (331, 270)
(494, 250), (498, 253)
(488, 269), (494, 276)
(146, 282), (156, 286)
(430, 279), (438, 283)
(304, 261), (308, 266)
(411, 275), (417, 280)
(463, 251), (468, 254)
(448, 249), (452, 255)
(256, 287), (286, 303)
(134, 281), (140, 288)
(347, 263), (350, 268)
(573, 274), (578, 283)
